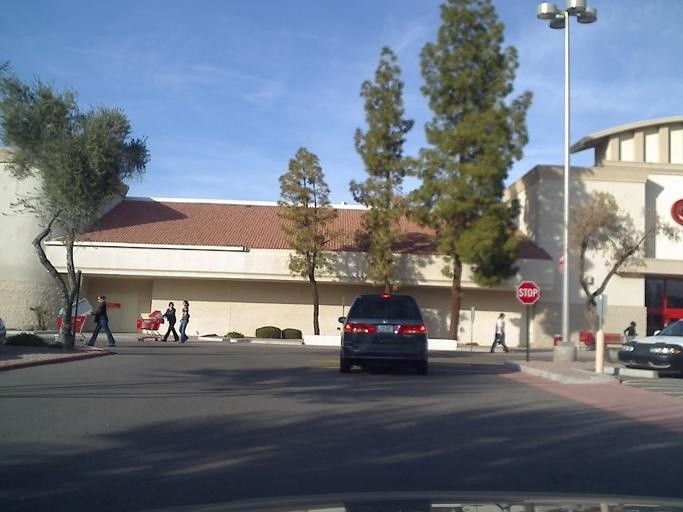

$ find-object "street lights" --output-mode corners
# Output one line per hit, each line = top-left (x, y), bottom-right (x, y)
(536, 0), (597, 363)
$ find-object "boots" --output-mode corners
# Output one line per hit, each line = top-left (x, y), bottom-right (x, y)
(161, 336), (189, 343)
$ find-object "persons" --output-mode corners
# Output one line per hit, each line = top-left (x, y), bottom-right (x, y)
(491, 313), (509, 353)
(163, 302), (179, 342)
(179, 300), (189, 343)
(624, 321), (638, 336)
(87, 295), (116, 346)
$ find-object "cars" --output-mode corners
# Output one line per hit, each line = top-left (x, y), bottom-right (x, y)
(338, 293), (428, 374)
(618, 318), (683, 371)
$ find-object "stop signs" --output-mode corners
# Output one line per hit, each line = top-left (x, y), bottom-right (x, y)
(516, 281), (541, 304)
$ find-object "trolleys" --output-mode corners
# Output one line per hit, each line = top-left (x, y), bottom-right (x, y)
(55, 297), (93, 344)
(137, 311), (164, 342)
